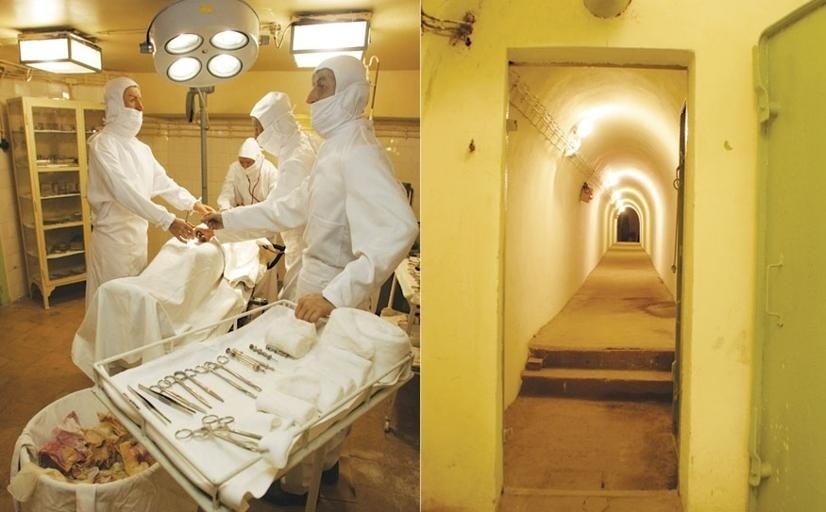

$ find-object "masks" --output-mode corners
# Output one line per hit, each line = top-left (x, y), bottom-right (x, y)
(245, 162), (258, 178)
(310, 86), (354, 135)
(256, 121), (288, 157)
(118, 107), (143, 133)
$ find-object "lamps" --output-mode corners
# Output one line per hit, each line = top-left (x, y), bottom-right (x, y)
(138, 0), (261, 88)
(291, 18), (370, 69)
(18, 28), (103, 75)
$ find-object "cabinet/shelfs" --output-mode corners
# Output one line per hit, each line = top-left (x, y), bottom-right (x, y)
(6, 96), (106, 310)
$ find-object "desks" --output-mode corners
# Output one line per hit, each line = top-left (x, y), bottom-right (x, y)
(380, 256), (420, 375)
(91, 299), (414, 512)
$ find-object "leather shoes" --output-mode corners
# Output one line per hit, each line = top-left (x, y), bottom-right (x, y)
(263, 479), (307, 507)
(322, 463), (339, 484)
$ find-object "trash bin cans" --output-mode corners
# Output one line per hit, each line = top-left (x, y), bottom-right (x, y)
(7, 388), (163, 512)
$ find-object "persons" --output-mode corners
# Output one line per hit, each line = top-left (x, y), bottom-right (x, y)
(250, 90), (316, 298)
(86, 75), (217, 308)
(216, 137), (280, 323)
(197, 55), (420, 506)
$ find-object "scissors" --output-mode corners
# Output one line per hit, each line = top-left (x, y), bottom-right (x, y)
(150, 355), (262, 414)
